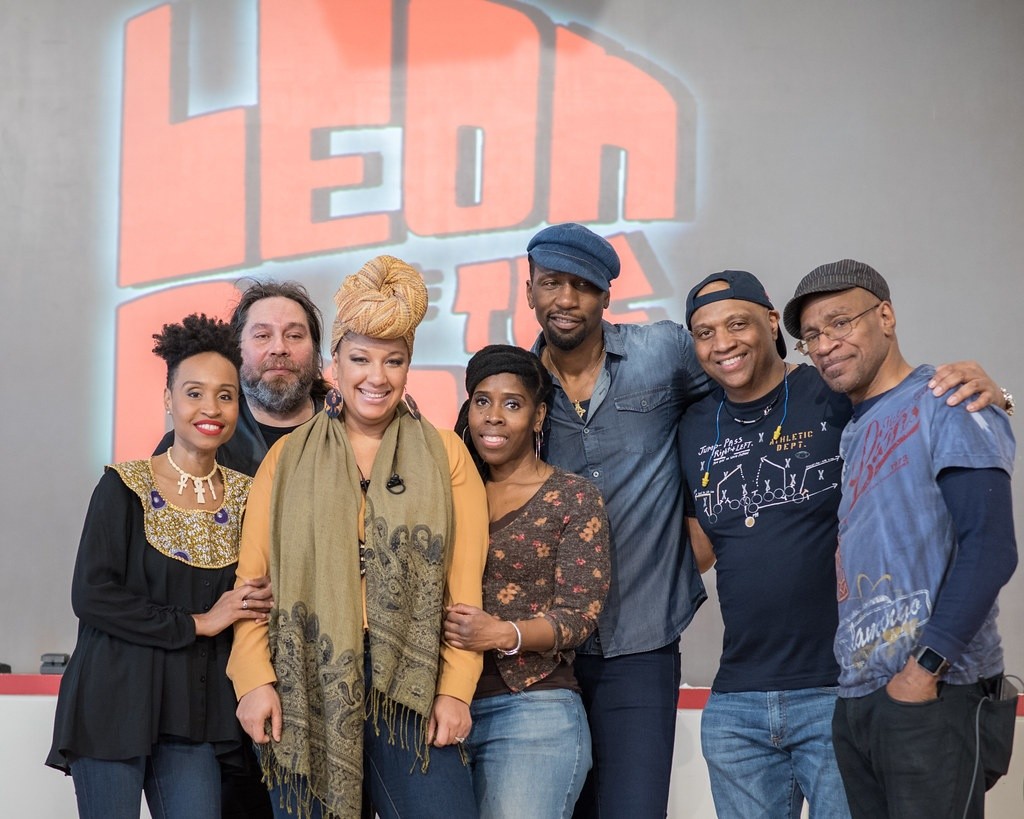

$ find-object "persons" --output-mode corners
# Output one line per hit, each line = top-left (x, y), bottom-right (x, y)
(782, 258), (1018, 819)
(457, 223), (729, 819)
(44, 315), (277, 819)
(436, 339), (610, 819)
(144, 279), (342, 819)
(683, 268), (1024, 818)
(221, 254), (490, 819)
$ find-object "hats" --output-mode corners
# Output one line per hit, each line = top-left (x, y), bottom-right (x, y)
(525, 222), (622, 290)
(686, 270), (788, 359)
(784, 258), (891, 339)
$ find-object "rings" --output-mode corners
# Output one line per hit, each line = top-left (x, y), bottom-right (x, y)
(243, 598), (248, 610)
(455, 735), (465, 744)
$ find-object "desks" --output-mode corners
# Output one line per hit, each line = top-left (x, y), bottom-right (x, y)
(0, 673), (811, 819)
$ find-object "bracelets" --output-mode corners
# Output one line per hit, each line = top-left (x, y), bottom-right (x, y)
(497, 620), (522, 656)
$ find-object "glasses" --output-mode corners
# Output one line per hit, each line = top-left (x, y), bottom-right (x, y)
(793, 304), (880, 356)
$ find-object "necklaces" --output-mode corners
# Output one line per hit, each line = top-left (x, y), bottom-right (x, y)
(166, 442), (220, 505)
(547, 346), (606, 419)
(723, 360), (789, 425)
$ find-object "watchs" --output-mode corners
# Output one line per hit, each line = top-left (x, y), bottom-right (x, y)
(999, 386), (1015, 415)
(908, 644), (952, 678)
